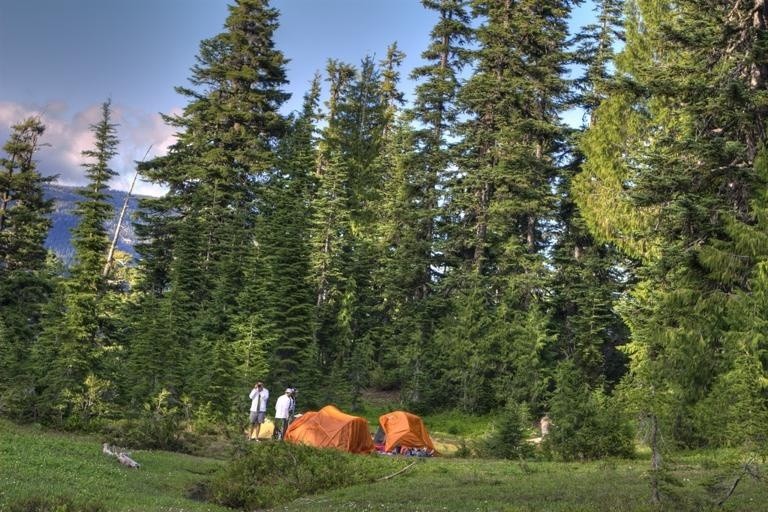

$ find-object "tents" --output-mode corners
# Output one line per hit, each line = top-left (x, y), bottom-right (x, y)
(374, 411), (437, 456)
(283, 405), (376, 455)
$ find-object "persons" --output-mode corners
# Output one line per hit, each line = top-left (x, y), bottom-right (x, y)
(281, 387), (298, 437)
(248, 381), (269, 441)
(540, 414), (555, 436)
(271, 388), (293, 441)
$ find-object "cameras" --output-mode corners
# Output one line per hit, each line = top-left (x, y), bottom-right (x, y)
(258, 385), (262, 387)
